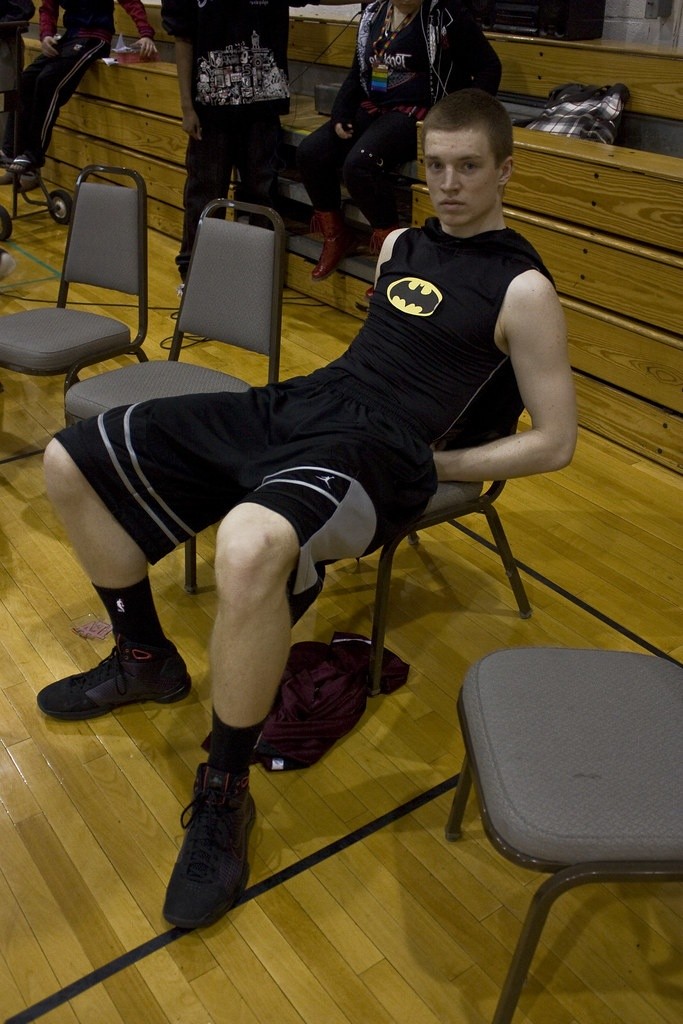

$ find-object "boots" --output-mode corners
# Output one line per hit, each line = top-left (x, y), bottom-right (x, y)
(310, 210), (359, 280)
(366, 225), (401, 299)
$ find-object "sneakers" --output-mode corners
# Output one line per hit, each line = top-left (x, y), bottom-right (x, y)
(163, 763), (256, 928)
(37, 630), (191, 720)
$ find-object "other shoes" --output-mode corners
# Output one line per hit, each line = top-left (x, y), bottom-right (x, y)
(0, 252), (16, 281)
(16, 174), (41, 193)
(0, 171), (14, 185)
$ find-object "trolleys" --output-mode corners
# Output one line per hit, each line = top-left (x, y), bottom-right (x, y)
(0, 20), (72, 242)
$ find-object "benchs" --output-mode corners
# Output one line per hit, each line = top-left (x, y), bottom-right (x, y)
(20, 0), (683, 474)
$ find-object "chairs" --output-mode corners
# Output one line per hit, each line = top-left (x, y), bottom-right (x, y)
(0, 164), (148, 396)
(446, 648), (682, 1024)
(370, 400), (534, 696)
(66, 200), (288, 592)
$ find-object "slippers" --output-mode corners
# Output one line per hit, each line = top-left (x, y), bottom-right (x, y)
(7, 155), (36, 174)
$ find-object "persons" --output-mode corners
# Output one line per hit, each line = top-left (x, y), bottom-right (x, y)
(0, 0), (502, 300)
(35, 89), (574, 924)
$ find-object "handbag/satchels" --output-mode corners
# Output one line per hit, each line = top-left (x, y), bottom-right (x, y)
(525, 84), (630, 145)
(201, 631), (409, 772)
